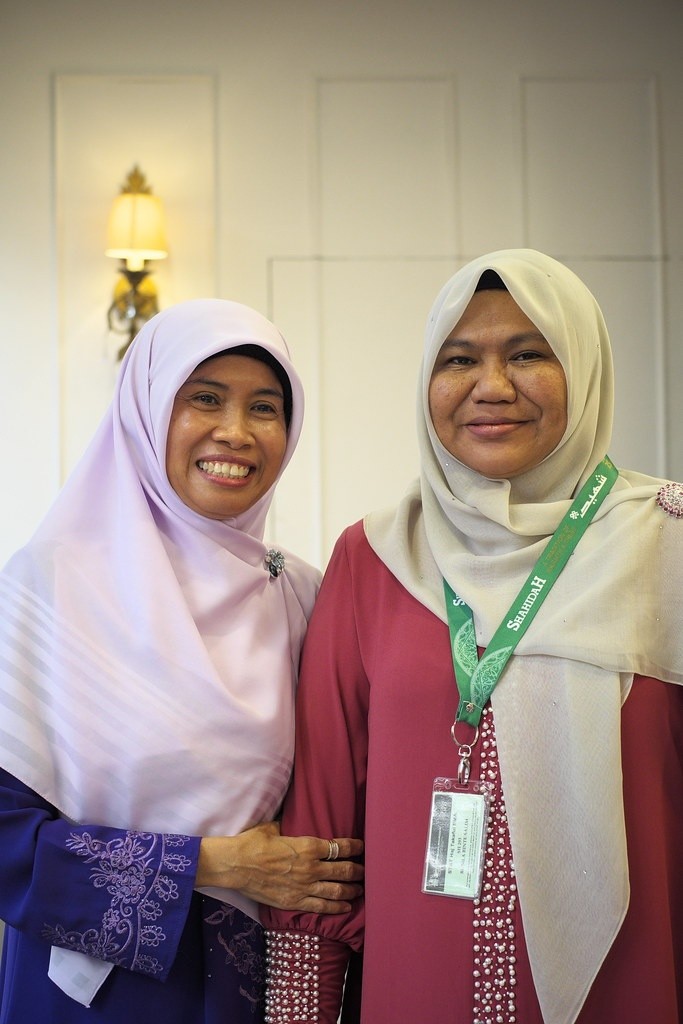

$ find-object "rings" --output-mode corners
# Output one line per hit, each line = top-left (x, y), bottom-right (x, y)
(328, 839), (339, 860)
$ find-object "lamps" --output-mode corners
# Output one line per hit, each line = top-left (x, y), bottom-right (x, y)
(108, 162), (168, 361)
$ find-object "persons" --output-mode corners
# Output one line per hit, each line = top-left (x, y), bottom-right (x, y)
(0, 297), (366, 1024)
(261, 247), (682, 1023)
